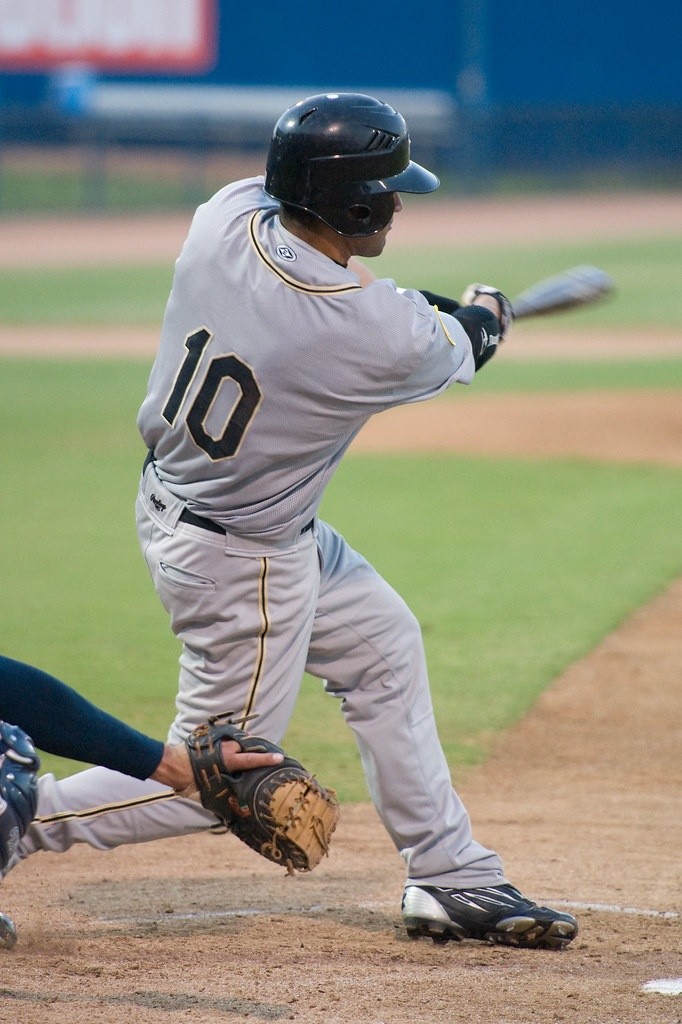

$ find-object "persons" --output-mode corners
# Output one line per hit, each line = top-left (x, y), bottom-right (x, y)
(0, 92), (579, 949)
(0, 656), (282, 948)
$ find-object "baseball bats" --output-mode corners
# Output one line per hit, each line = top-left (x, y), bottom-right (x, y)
(506, 262), (613, 322)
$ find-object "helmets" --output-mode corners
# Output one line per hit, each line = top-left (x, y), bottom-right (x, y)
(262, 92), (442, 239)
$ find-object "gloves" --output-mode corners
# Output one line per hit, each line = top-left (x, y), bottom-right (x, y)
(463, 283), (513, 341)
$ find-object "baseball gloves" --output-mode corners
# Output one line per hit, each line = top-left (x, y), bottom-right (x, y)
(180, 724), (343, 877)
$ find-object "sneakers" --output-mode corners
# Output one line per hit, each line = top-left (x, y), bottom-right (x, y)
(400, 883), (577, 950)
